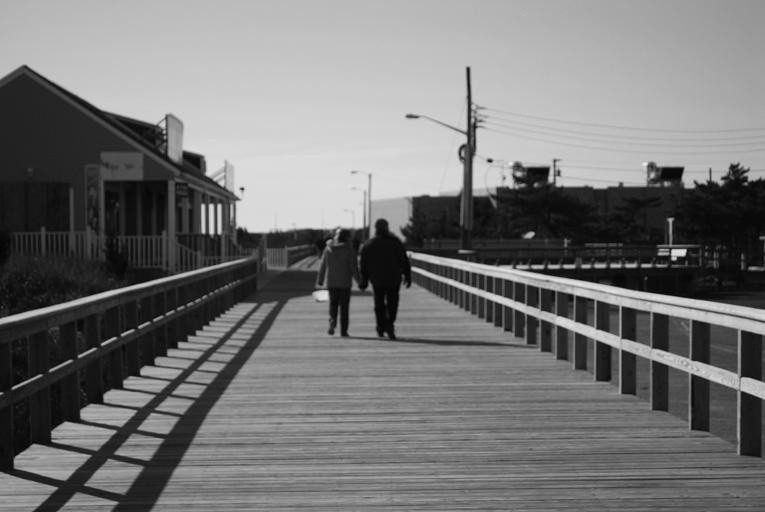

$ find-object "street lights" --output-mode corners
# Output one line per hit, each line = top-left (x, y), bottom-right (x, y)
(405, 66), (476, 250)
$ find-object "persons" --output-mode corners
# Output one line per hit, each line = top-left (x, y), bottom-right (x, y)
(358, 217), (412, 339)
(315, 227), (365, 338)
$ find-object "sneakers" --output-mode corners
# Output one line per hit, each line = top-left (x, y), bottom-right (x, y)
(328, 317), (348, 336)
(376, 324), (397, 341)
